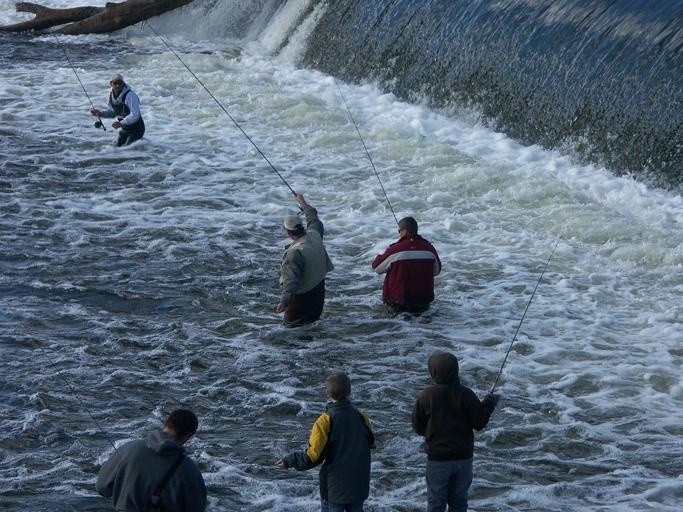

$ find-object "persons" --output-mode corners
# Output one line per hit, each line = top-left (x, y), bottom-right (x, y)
(90, 74), (145, 147)
(95, 410), (208, 512)
(275, 191), (335, 327)
(274, 372), (376, 512)
(412, 350), (502, 512)
(371, 216), (441, 314)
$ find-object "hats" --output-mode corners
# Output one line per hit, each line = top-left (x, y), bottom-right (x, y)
(283, 215), (301, 231)
(111, 74), (122, 85)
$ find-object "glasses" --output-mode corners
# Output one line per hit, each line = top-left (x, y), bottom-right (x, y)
(398, 229), (403, 233)
